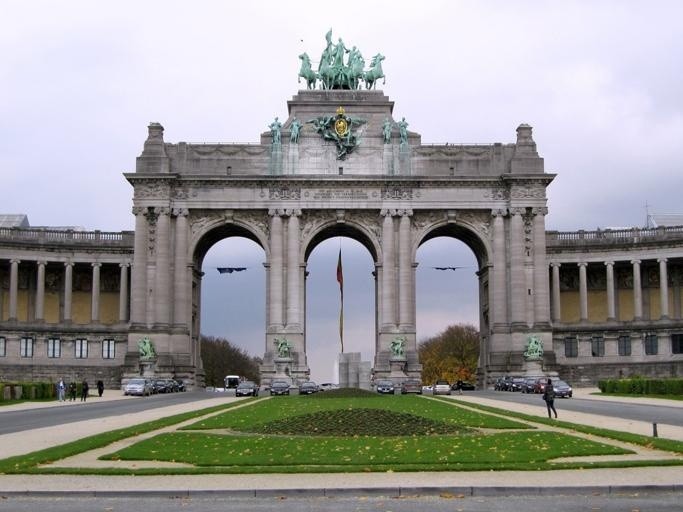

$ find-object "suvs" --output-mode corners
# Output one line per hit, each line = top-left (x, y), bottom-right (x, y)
(400, 380), (422, 394)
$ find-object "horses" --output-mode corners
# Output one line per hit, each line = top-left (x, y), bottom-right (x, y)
(318, 51), (340, 91)
(298, 52), (320, 90)
(346, 50), (365, 90)
(362, 53), (386, 90)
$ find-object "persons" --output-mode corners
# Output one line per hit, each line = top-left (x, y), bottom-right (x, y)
(332, 37), (347, 66)
(457, 378), (463, 394)
(55, 377), (104, 402)
(136, 336), (155, 356)
(272, 335), (290, 358)
(268, 114), (409, 150)
(542, 378), (558, 418)
(346, 46), (359, 64)
(387, 336), (409, 357)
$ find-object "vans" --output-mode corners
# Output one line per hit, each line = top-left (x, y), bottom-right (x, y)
(318, 382), (341, 392)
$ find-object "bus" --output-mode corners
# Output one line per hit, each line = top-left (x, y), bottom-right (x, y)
(224, 375), (248, 392)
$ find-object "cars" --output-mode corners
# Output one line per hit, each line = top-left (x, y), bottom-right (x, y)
(433, 380), (451, 394)
(452, 382), (475, 390)
(124, 377), (186, 396)
(494, 375), (573, 399)
(298, 382), (319, 394)
(377, 380), (394, 394)
(235, 381), (260, 397)
(270, 381), (290, 395)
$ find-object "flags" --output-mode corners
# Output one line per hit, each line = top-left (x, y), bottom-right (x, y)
(336, 249), (342, 290)
